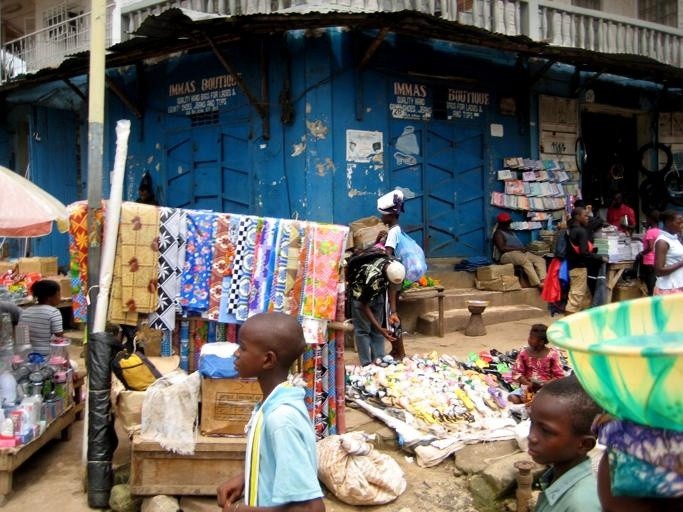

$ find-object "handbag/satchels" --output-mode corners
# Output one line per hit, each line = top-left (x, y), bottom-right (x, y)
(345, 252), (389, 282)
(572, 240), (593, 254)
(111, 351), (163, 391)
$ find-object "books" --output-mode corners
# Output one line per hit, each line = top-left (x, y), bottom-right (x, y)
(564, 183), (582, 200)
(490, 183), (547, 231)
(497, 157), (582, 183)
(546, 183), (564, 230)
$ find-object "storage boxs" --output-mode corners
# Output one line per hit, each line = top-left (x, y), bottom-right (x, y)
(201, 377), (264, 438)
(42, 276), (72, 297)
(612, 285), (641, 301)
(130, 434), (245, 497)
(0, 257), (19, 275)
(19, 257), (58, 276)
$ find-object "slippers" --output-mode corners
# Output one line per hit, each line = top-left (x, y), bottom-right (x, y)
(481, 389), (498, 410)
(387, 408), (476, 438)
(468, 389), (485, 412)
(455, 389), (474, 411)
(345, 351), (455, 408)
(455, 349), (519, 389)
(488, 386), (505, 407)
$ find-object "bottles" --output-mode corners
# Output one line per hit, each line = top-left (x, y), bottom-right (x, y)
(11, 411), (24, 431)
(17, 354), (57, 399)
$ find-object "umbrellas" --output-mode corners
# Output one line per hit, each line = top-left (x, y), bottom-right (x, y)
(0, 163), (71, 239)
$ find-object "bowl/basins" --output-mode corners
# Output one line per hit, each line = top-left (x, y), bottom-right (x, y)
(544, 293), (683, 432)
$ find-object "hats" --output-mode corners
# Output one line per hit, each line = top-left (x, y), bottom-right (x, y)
(387, 262), (405, 285)
(497, 213), (513, 224)
(378, 190), (405, 215)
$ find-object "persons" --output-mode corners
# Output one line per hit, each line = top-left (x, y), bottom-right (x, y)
(567, 200), (594, 244)
(590, 410), (683, 511)
(652, 209), (683, 296)
(373, 190), (405, 256)
(526, 376), (602, 512)
(607, 193), (637, 236)
(216, 312), (327, 512)
(0, 300), (25, 326)
(564, 207), (609, 316)
(508, 324), (564, 404)
(637, 210), (661, 295)
(346, 254), (406, 368)
(15, 280), (81, 374)
(82, 323), (87, 371)
(492, 212), (547, 288)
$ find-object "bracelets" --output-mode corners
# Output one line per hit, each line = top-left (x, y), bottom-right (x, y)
(389, 310), (397, 315)
(233, 503), (240, 512)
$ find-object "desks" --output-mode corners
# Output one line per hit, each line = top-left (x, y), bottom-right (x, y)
(399, 284), (446, 338)
(0, 402), (76, 495)
(607, 261), (647, 303)
